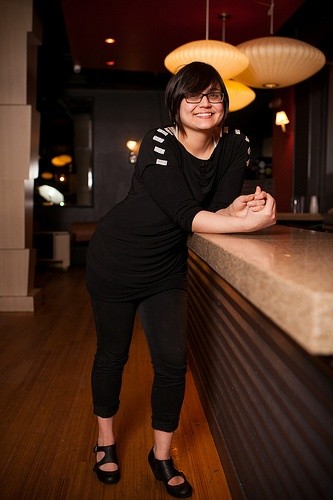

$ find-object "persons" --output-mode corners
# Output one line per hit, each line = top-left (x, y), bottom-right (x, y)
(85, 61), (277, 497)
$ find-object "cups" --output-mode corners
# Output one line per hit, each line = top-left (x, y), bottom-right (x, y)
(310, 197), (318, 214)
(293, 197), (303, 214)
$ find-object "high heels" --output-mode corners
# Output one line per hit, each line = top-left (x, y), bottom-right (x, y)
(148, 447), (192, 497)
(94, 442), (121, 485)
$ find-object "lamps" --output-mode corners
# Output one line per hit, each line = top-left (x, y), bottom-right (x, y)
(218, 13), (255, 113)
(276, 111), (290, 132)
(233, 0), (325, 88)
(164, 0), (250, 80)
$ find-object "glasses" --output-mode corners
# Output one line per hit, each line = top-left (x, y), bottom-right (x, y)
(183, 92), (225, 104)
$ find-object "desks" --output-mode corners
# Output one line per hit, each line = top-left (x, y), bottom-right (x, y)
(44, 220), (98, 241)
